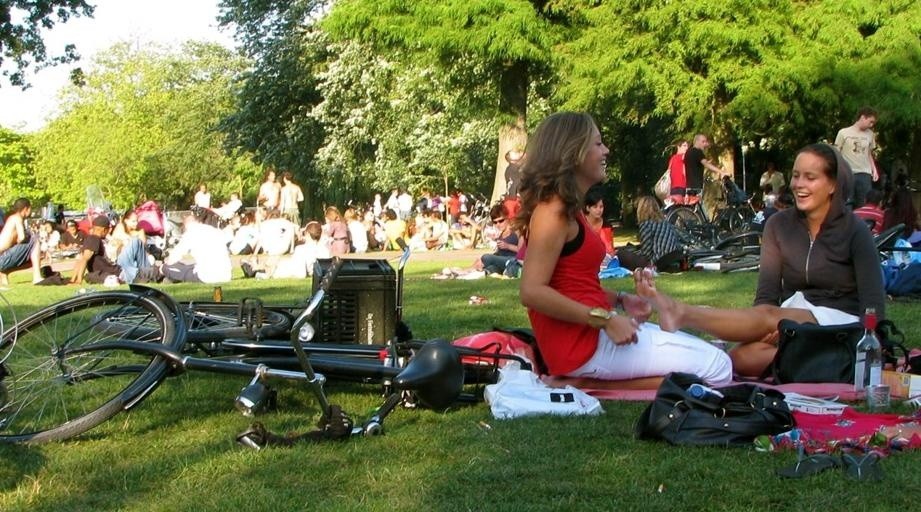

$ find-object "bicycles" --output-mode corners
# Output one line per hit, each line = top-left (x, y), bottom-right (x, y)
(665, 175), (769, 271)
(467, 190), (491, 221)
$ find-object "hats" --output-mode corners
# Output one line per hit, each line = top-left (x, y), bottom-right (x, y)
(92, 215), (111, 228)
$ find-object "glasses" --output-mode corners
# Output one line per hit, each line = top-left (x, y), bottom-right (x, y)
(493, 219), (506, 225)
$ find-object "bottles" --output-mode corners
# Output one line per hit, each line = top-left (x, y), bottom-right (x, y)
(683, 383), (713, 399)
(853, 307), (881, 393)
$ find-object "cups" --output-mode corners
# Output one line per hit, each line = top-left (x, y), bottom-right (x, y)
(867, 383), (891, 411)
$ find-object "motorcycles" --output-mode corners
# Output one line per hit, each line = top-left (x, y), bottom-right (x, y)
(85, 183), (117, 226)
